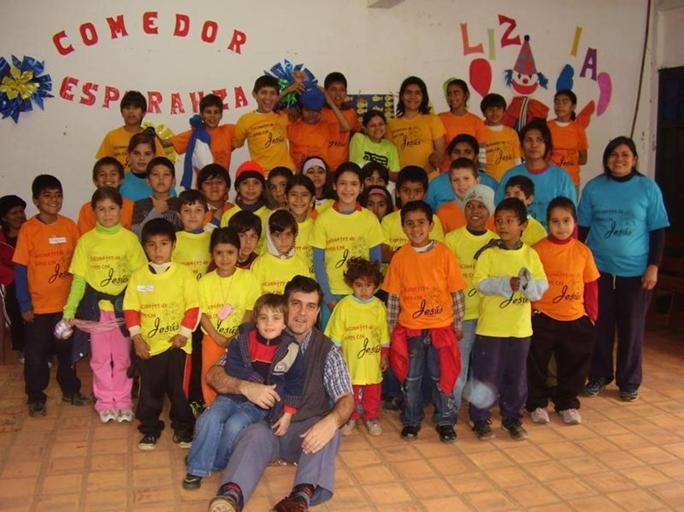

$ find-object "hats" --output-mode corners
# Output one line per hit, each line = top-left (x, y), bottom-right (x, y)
(463, 185), (495, 220)
(299, 85), (324, 110)
(234, 160), (264, 190)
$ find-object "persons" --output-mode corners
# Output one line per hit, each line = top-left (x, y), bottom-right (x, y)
(171, 191), (217, 277)
(267, 166), (292, 207)
(471, 197), (549, 441)
(426, 130), (498, 208)
(56, 187), (148, 424)
(545, 90), (589, 184)
(133, 157), (179, 236)
(222, 161), (271, 254)
(323, 259), (390, 436)
(438, 157), (495, 233)
(250, 209), (317, 294)
(504, 175), (547, 245)
(477, 92), (523, 182)
(79, 156), (134, 233)
(197, 163), (233, 228)
(288, 88), (349, 169)
(529, 195), (600, 425)
(122, 217), (201, 450)
(349, 110), (400, 182)
(361, 162), (388, 187)
(162, 94), (236, 189)
(119, 126), (175, 202)
(0, 194), (28, 363)
(494, 117), (579, 236)
(96, 90), (167, 174)
(323, 72), (361, 135)
(231, 75), (296, 174)
(281, 174), (316, 259)
(198, 227), (262, 407)
(182, 294), (306, 489)
(13, 175), (90, 419)
(382, 201), (468, 443)
(362, 186), (392, 216)
(438, 80), (483, 145)
(207, 208), (261, 270)
(382, 165), (444, 260)
(577, 135), (670, 402)
(209, 276), (355, 512)
(309, 161), (380, 329)
(386, 75), (446, 180)
(446, 185), (502, 411)
(300, 157), (329, 199)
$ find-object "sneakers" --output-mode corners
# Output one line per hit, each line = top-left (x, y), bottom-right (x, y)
(17, 351), (25, 363)
(434, 424), (456, 443)
(530, 407), (549, 424)
(273, 497), (307, 512)
(341, 417), (356, 436)
(28, 401), (45, 416)
(63, 393), (89, 406)
(619, 388), (638, 401)
(181, 474), (202, 488)
(582, 379), (605, 396)
(116, 407), (132, 423)
(383, 396), (399, 410)
(401, 421), (421, 439)
(366, 416), (381, 436)
(208, 494), (239, 511)
(558, 408), (581, 425)
(470, 419), (496, 440)
(500, 415), (527, 440)
(137, 435), (157, 449)
(98, 407), (116, 423)
(171, 432), (192, 448)
